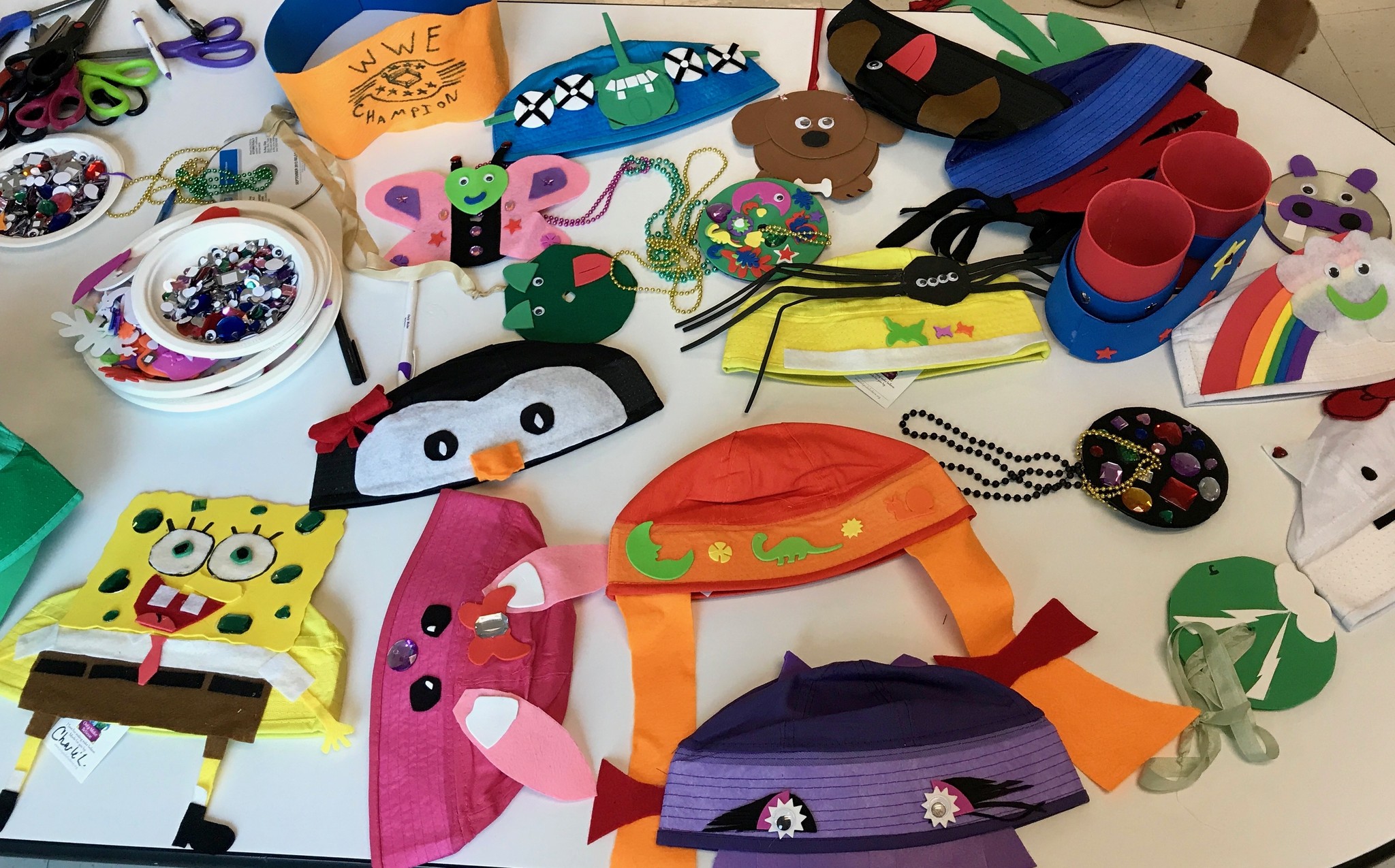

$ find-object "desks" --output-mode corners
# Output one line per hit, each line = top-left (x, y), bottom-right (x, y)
(0, 0), (1395, 868)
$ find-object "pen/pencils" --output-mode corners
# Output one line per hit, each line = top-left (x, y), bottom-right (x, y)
(156, 0), (210, 46)
(154, 188), (177, 227)
(335, 315), (366, 388)
(130, 9), (172, 80)
(399, 278), (420, 387)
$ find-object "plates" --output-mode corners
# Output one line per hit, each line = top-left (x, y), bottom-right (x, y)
(79, 201), (342, 414)
(0, 132), (126, 246)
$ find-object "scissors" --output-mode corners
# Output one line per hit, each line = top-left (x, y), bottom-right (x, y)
(0, 0), (157, 150)
(78, 16), (256, 69)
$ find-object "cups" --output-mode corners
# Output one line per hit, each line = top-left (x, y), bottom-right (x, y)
(1075, 177), (1194, 301)
(1155, 130), (1273, 238)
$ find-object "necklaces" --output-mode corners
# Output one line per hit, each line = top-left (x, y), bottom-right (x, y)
(900, 410), (1162, 504)
(543, 146), (728, 314)
(107, 145), (277, 218)
